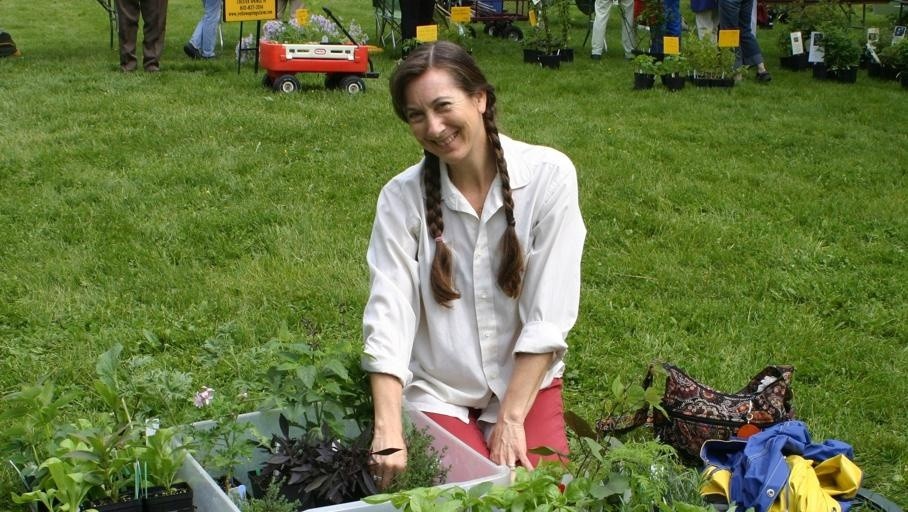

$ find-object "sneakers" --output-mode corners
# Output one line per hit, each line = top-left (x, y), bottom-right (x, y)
(624, 49), (665, 66)
(590, 52), (602, 62)
(755, 68), (772, 82)
(183, 41), (216, 63)
(119, 60), (163, 74)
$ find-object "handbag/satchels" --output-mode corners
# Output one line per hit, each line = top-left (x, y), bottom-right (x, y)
(597, 357), (797, 470)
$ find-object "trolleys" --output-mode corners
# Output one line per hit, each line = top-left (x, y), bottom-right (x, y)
(258, 7), (380, 98)
(434, 0), (531, 43)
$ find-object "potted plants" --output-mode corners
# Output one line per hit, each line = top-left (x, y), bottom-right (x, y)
(631, 52), (660, 88)
(685, 32), (750, 87)
(777, 0), (907, 88)
(630, 0), (676, 61)
(11, 351), (193, 512)
(250, 334), (452, 511)
(522, 0), (574, 71)
(656, 54), (691, 90)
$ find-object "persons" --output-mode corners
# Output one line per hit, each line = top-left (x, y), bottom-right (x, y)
(183, 0), (224, 59)
(398, 0), (435, 64)
(590, 0), (771, 84)
(115, 0), (167, 73)
(276, 0), (306, 21)
(359, 40), (587, 493)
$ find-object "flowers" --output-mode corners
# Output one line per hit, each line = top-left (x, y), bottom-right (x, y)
(263, 15), (370, 46)
(169, 382), (256, 492)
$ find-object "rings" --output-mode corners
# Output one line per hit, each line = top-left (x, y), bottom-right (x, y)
(373, 475), (382, 480)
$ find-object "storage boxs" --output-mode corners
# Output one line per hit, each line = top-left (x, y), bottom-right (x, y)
(166, 392), (512, 512)
